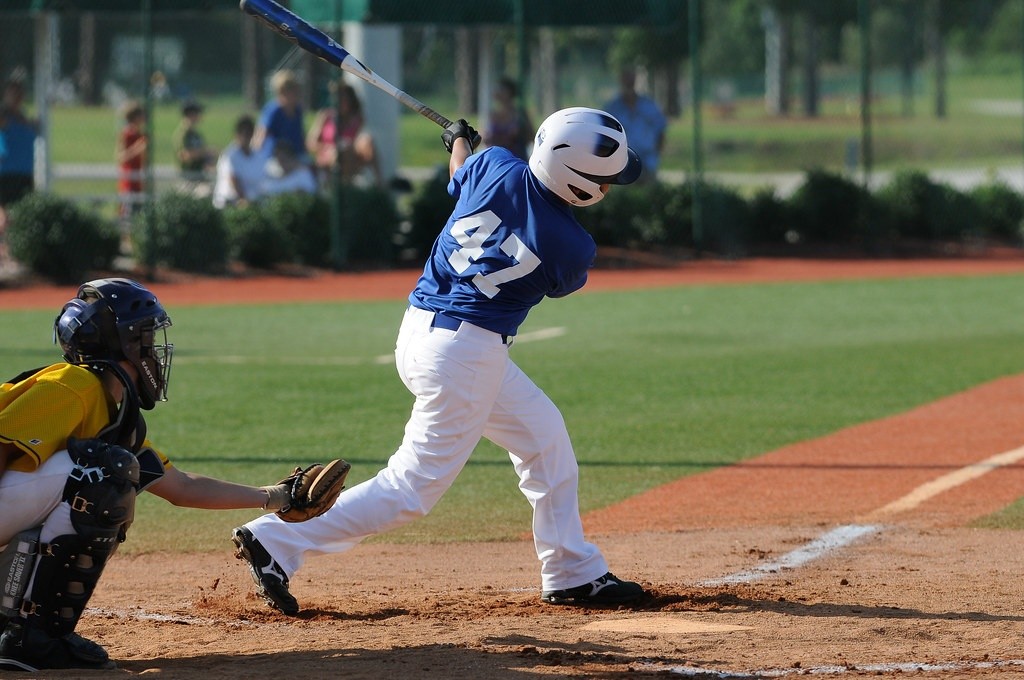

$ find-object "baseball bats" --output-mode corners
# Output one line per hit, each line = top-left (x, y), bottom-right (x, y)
(238, 0), (475, 140)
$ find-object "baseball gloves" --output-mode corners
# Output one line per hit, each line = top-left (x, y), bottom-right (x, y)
(274, 458), (356, 528)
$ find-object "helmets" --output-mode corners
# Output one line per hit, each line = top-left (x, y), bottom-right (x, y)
(53, 277), (173, 365)
(529, 105), (643, 207)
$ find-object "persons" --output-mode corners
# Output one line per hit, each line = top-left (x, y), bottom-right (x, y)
(479, 63), (667, 196)
(0, 279), (350, 672)
(104, 66), (385, 259)
(233, 108), (642, 618)
(0, 78), (42, 210)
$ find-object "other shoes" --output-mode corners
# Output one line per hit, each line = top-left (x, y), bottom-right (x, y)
(0, 630), (109, 672)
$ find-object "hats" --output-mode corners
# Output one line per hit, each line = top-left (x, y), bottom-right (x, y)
(271, 70), (303, 93)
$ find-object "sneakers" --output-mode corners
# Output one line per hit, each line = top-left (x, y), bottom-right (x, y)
(540, 572), (644, 607)
(232, 525), (300, 617)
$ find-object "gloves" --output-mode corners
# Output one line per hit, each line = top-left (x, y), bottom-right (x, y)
(441, 119), (482, 154)
